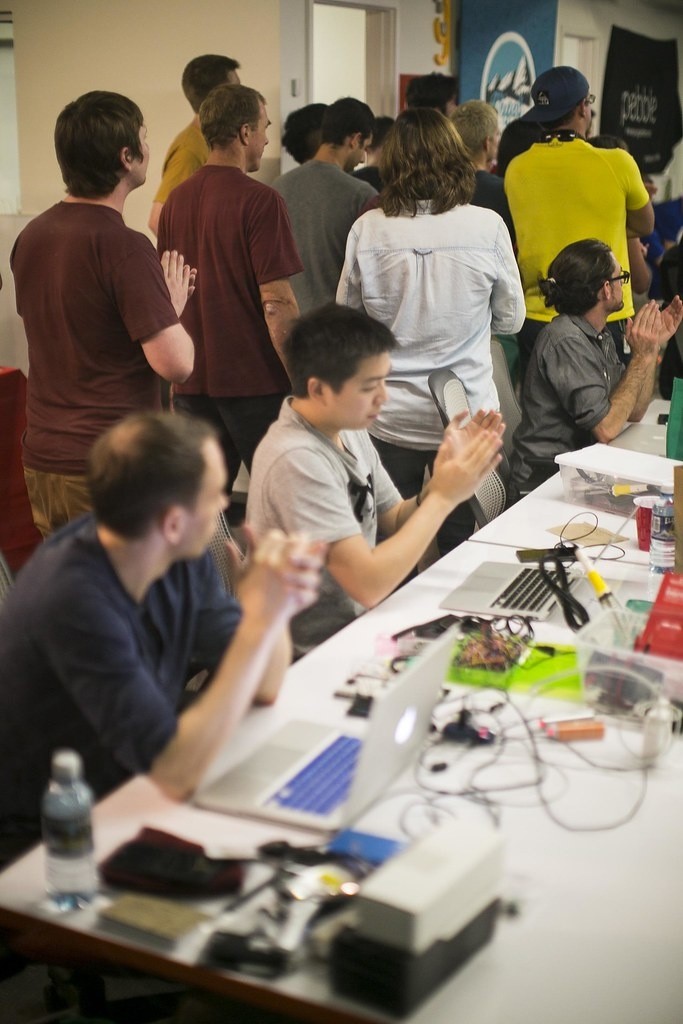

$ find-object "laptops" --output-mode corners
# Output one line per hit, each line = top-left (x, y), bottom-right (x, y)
(440, 499), (645, 623)
(193, 623), (462, 832)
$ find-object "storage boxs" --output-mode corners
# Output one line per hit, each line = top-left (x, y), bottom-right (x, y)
(554, 443), (683, 520)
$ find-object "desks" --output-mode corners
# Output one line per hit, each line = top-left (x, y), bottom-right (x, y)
(0, 365), (44, 571)
(0, 399), (683, 1024)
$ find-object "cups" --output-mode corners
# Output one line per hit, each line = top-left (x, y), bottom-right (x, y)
(633, 495), (660, 552)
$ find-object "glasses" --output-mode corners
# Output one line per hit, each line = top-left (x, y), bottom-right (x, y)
(609, 271), (629, 284)
(588, 95), (596, 104)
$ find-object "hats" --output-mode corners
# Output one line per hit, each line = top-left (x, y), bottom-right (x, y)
(522, 66), (590, 122)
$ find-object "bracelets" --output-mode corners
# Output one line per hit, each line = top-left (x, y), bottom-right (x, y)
(416, 492), (423, 506)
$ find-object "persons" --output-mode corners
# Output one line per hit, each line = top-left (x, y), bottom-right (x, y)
(450, 99), (516, 244)
(1, 408), (330, 873)
(498, 108), (683, 424)
(334, 108), (527, 591)
(155, 83), (303, 495)
(503, 66), (655, 368)
(9, 90), (197, 541)
(282, 103), (328, 165)
(407, 75), (460, 116)
(245, 303), (506, 665)
(350, 116), (395, 195)
(509, 238), (682, 504)
(148, 55), (241, 235)
(268, 97), (379, 322)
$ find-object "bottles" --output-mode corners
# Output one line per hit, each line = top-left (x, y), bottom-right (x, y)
(648, 482), (675, 573)
(42, 750), (97, 913)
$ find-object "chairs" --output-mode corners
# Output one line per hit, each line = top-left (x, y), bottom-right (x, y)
(0, 504), (245, 696)
(426, 336), (525, 529)
